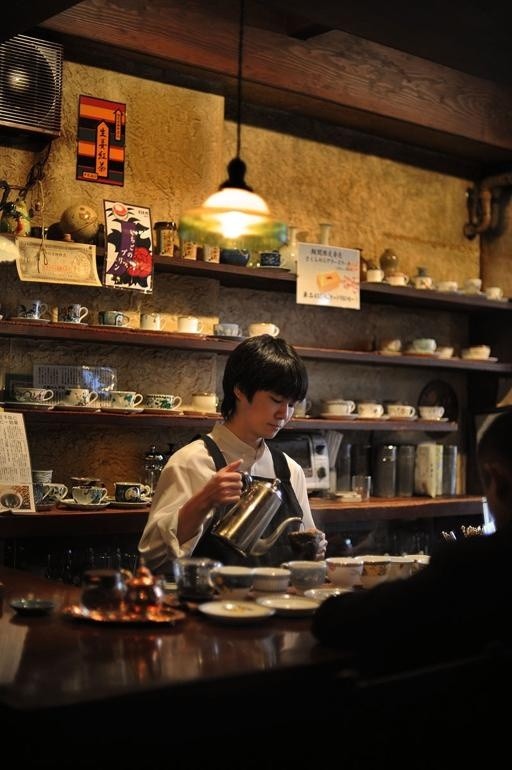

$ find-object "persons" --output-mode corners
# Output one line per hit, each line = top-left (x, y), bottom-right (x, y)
(136, 334), (330, 586)
(302, 401), (512, 769)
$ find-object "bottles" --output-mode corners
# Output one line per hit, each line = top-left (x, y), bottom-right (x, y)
(379, 248), (399, 273)
(280, 226), (301, 269)
(141, 442), (178, 490)
(345, 441), (472, 503)
(317, 224), (331, 245)
(125, 569), (164, 617)
(355, 248), (368, 279)
(175, 562), (205, 609)
(83, 574), (123, 612)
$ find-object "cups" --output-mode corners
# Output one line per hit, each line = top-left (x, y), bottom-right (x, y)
(248, 323), (281, 339)
(328, 557), (364, 585)
(289, 529), (322, 561)
(153, 221), (220, 263)
(392, 555), (416, 577)
(295, 399), (445, 419)
(416, 557), (431, 574)
(284, 560), (327, 587)
(189, 392), (219, 413)
(16, 299), (88, 322)
(6, 542), (139, 585)
(30, 466), (151, 502)
(254, 566), (291, 592)
(206, 565), (254, 598)
(212, 323), (243, 336)
(259, 253), (281, 267)
(362, 557), (392, 586)
(365, 268), (505, 301)
(98, 309), (130, 327)
(379, 337), (500, 362)
(139, 314), (201, 334)
(12, 386), (182, 410)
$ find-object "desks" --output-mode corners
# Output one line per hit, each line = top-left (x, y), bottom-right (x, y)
(1, 554), (449, 716)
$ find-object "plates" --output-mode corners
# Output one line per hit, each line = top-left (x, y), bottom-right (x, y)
(260, 593), (316, 610)
(13, 596), (58, 613)
(184, 409), (222, 417)
(0, 401), (183, 416)
(32, 500), (149, 512)
(11, 617), (56, 631)
(256, 267), (292, 273)
(291, 413), (447, 423)
(304, 584), (350, 602)
(198, 596), (270, 618)
(91, 324), (134, 333)
(12, 317), (90, 328)
(207, 334), (244, 342)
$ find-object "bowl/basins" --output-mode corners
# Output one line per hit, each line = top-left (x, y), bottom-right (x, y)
(0, 489), (23, 509)
(221, 248), (250, 265)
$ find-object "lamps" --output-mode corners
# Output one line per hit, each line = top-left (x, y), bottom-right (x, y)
(181, 1), (290, 240)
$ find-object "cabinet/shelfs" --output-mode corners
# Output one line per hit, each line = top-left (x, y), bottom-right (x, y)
(1, 231), (512, 529)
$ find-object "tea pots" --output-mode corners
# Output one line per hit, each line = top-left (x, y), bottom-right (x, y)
(210, 469), (305, 559)
